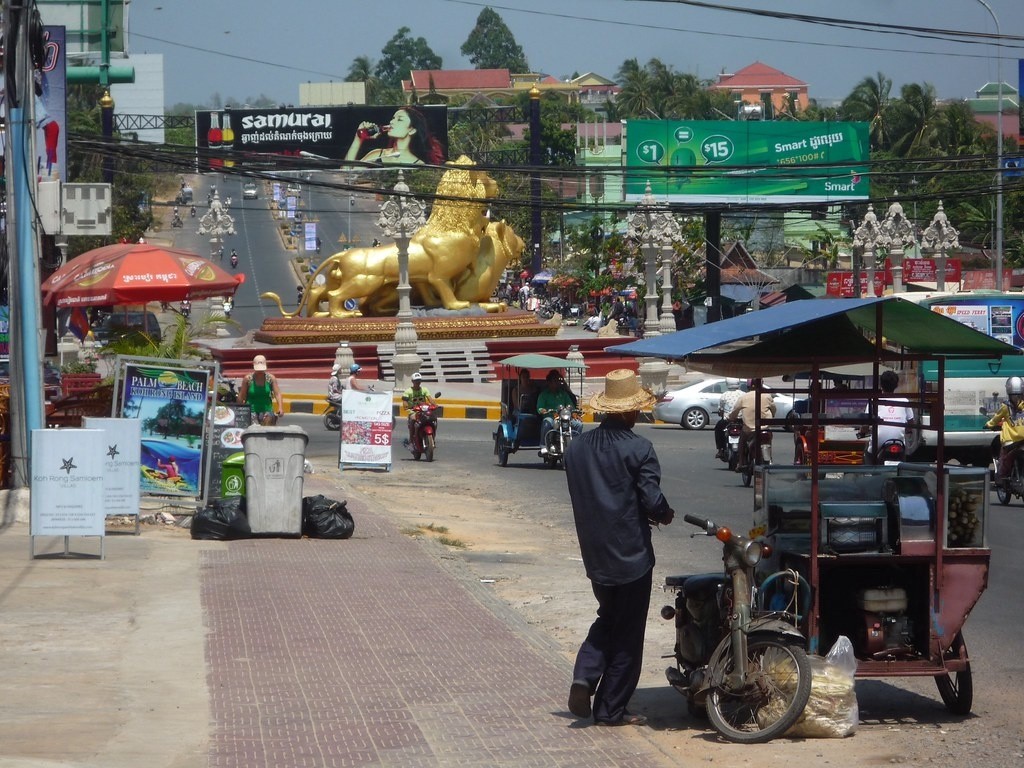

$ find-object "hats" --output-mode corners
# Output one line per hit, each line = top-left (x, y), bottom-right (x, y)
(350, 364), (360, 373)
(252, 355), (267, 371)
(330, 364), (341, 375)
(411, 373), (422, 381)
(589, 368), (656, 414)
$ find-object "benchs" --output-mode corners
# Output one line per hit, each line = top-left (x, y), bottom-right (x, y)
(500, 379), (545, 419)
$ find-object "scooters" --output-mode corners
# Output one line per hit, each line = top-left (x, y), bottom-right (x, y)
(224, 303), (232, 314)
(180, 300), (191, 318)
(171, 219), (183, 228)
(231, 255), (238, 268)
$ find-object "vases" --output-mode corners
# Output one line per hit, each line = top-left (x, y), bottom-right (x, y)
(61, 373), (101, 395)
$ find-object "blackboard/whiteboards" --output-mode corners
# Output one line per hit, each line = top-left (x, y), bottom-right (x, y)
(219, 452), (246, 500)
(203, 426), (245, 497)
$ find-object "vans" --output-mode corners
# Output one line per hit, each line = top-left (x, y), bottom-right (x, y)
(287, 183), (301, 198)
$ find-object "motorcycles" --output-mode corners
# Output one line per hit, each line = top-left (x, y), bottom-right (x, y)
(782, 363), (904, 467)
(402, 392), (443, 462)
(218, 373), (239, 403)
(979, 408), (1024, 505)
(660, 515), (812, 743)
(711, 411), (773, 487)
(322, 384), (396, 431)
(603, 296), (1024, 714)
(492, 354), (590, 471)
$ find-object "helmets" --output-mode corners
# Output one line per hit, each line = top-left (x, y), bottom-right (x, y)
(1004, 376), (1024, 396)
(725, 377), (741, 389)
(747, 378), (763, 387)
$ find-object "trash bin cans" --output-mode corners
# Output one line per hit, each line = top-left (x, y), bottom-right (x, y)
(240, 421), (308, 536)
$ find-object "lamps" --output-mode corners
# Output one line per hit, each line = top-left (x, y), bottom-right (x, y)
(568, 345), (579, 352)
(224, 104), (231, 112)
(339, 340), (349, 347)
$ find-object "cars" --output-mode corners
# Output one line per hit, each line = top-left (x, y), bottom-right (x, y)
(243, 184), (258, 199)
(43, 366), (63, 398)
(653, 376), (807, 432)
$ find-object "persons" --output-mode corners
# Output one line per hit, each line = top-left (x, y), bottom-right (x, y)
(296, 285), (304, 317)
(985, 375), (1024, 487)
(505, 280), (531, 306)
(149, 455), (179, 479)
(222, 296), (231, 306)
(803, 376), (847, 413)
(860, 370), (914, 465)
(172, 213), (183, 228)
(714, 377), (777, 473)
(230, 248), (237, 257)
(512, 368), (584, 455)
(350, 193), (356, 205)
(564, 368), (675, 727)
(583, 297), (637, 332)
(316, 238), (321, 247)
(340, 107), (432, 170)
(190, 204), (196, 213)
(327, 362), (371, 404)
(372, 237), (380, 247)
(402, 372), (438, 449)
(236, 355), (284, 426)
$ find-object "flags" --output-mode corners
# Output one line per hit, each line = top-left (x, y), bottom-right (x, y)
(68, 306), (91, 344)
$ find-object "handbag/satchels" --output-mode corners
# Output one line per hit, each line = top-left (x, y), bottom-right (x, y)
(754, 634), (861, 737)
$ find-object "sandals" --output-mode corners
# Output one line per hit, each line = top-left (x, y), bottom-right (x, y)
(594, 712), (647, 727)
(569, 680), (592, 719)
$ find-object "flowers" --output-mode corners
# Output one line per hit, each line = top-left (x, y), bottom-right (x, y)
(62, 344), (103, 374)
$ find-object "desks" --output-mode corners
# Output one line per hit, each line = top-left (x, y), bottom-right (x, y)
(51, 395), (112, 408)
(48, 408), (108, 425)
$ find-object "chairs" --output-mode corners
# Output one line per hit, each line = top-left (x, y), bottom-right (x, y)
(46, 384), (123, 427)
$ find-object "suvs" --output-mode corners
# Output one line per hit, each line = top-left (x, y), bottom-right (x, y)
(91, 311), (161, 349)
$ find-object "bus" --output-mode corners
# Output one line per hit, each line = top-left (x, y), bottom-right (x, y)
(887, 291), (1024, 468)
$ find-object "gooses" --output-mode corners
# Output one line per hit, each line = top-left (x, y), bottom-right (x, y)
(583, 308), (604, 332)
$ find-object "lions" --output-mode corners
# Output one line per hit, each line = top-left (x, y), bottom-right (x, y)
(260, 154), (526, 320)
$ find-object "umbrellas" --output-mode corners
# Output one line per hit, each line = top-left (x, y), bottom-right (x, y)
(520, 269), (558, 289)
(41, 241), (240, 335)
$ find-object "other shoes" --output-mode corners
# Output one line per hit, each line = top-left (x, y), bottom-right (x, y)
(540, 448), (547, 455)
(735, 464), (747, 472)
(715, 451), (725, 458)
